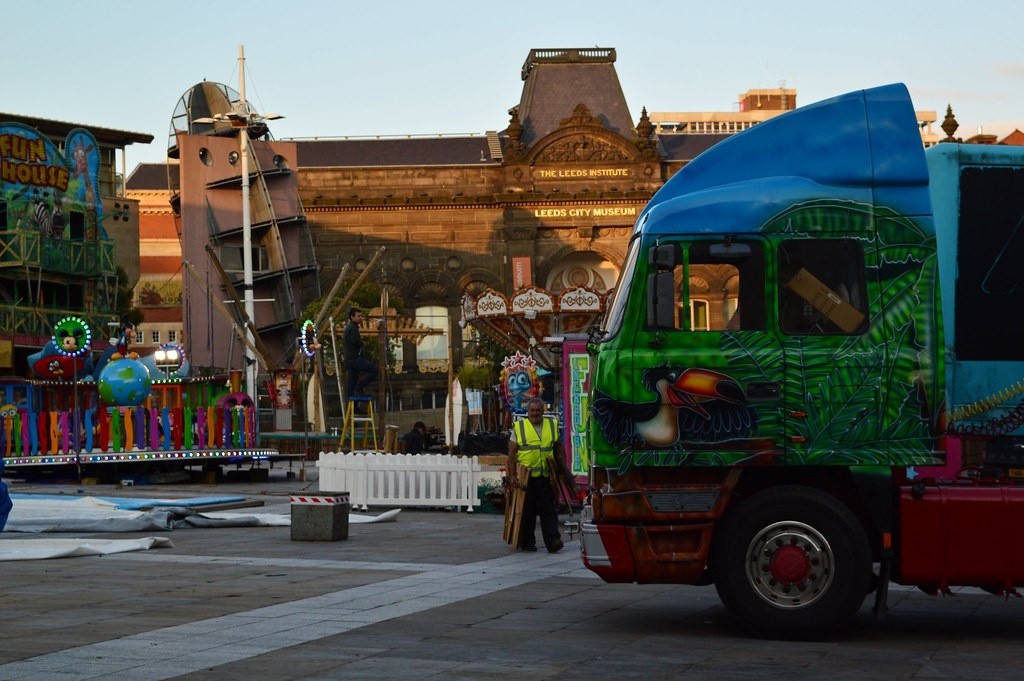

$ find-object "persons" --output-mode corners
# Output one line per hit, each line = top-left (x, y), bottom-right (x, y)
(508, 398), (564, 553)
(342, 308), (379, 397)
(402, 421), (426, 454)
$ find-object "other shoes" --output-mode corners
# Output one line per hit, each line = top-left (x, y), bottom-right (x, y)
(521, 544), (537, 552)
(354, 390), (371, 397)
(546, 538), (564, 553)
(354, 422), (360, 428)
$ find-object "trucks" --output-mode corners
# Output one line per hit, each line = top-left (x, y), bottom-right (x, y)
(578, 82), (1024, 637)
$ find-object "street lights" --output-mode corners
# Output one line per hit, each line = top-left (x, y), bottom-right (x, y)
(192, 44), (287, 447)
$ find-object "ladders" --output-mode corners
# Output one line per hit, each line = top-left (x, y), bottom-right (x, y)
(338, 394), (379, 453)
